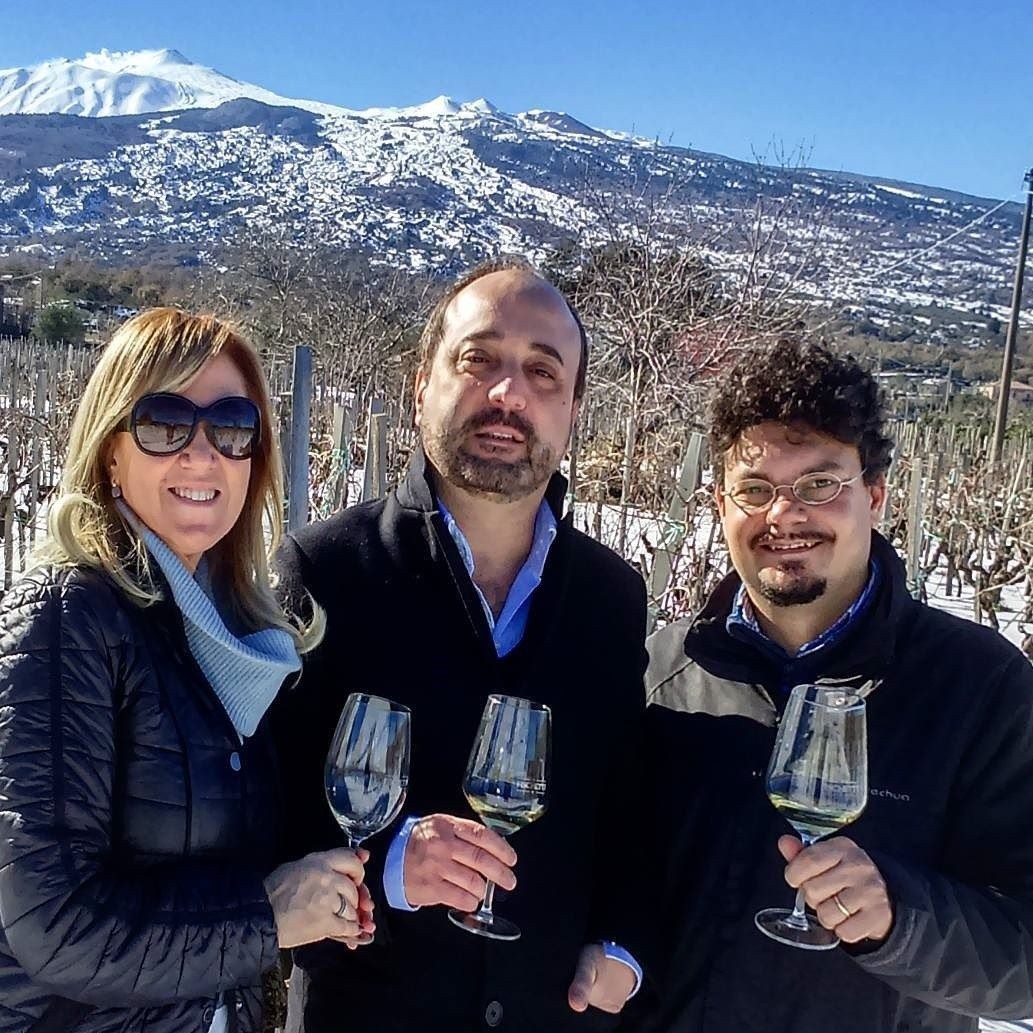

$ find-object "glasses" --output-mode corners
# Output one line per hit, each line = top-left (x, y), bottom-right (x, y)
(720, 465), (868, 510)
(115, 393), (261, 460)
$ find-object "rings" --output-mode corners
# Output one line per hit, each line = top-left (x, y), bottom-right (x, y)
(335, 891), (346, 917)
(834, 893), (851, 918)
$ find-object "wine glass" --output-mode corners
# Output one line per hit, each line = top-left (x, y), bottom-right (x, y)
(754, 682), (868, 951)
(323, 692), (415, 946)
(446, 693), (553, 943)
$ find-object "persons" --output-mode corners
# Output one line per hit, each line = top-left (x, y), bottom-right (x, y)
(646, 340), (1033, 1033)
(251, 269), (647, 1033)
(0, 304), (376, 1033)
(165, 420), (174, 445)
